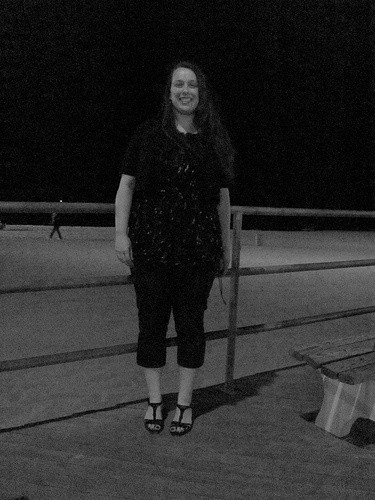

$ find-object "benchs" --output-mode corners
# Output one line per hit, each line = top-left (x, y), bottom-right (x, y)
(290, 330), (375, 438)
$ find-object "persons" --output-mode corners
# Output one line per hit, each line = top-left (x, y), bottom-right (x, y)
(49, 200), (65, 241)
(114, 60), (235, 436)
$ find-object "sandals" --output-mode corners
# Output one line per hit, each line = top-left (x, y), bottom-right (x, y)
(169, 400), (194, 434)
(144, 394), (165, 433)
